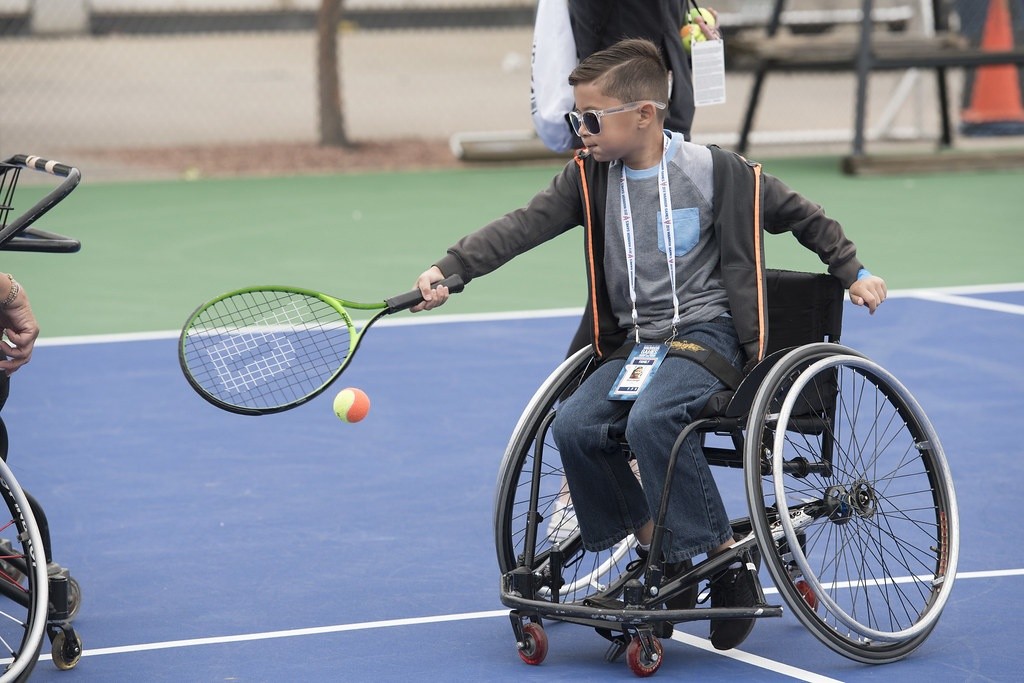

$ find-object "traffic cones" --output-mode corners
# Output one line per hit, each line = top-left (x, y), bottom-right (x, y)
(955, 0), (1024, 134)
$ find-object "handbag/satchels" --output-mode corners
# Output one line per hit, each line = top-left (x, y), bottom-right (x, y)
(530, 1), (579, 161)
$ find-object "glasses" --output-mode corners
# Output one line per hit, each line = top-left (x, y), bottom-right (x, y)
(568, 99), (666, 138)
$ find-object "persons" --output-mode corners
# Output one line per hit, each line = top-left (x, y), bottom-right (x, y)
(566, 0), (719, 150)
(0, 272), (40, 585)
(410, 41), (888, 651)
(630, 367), (643, 379)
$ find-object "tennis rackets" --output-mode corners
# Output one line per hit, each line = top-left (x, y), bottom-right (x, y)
(177, 274), (464, 416)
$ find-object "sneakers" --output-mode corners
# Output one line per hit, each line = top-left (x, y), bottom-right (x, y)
(625, 543), (700, 619)
(710, 535), (761, 652)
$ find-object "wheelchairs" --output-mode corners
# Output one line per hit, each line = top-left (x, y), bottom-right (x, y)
(0, 152), (82, 683)
(495, 269), (960, 679)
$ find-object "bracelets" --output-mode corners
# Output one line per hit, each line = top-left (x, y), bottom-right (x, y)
(0, 273), (20, 307)
(856, 269), (871, 280)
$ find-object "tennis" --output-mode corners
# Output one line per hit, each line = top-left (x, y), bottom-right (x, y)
(333, 387), (371, 423)
(679, 24), (708, 54)
(688, 7), (716, 30)
